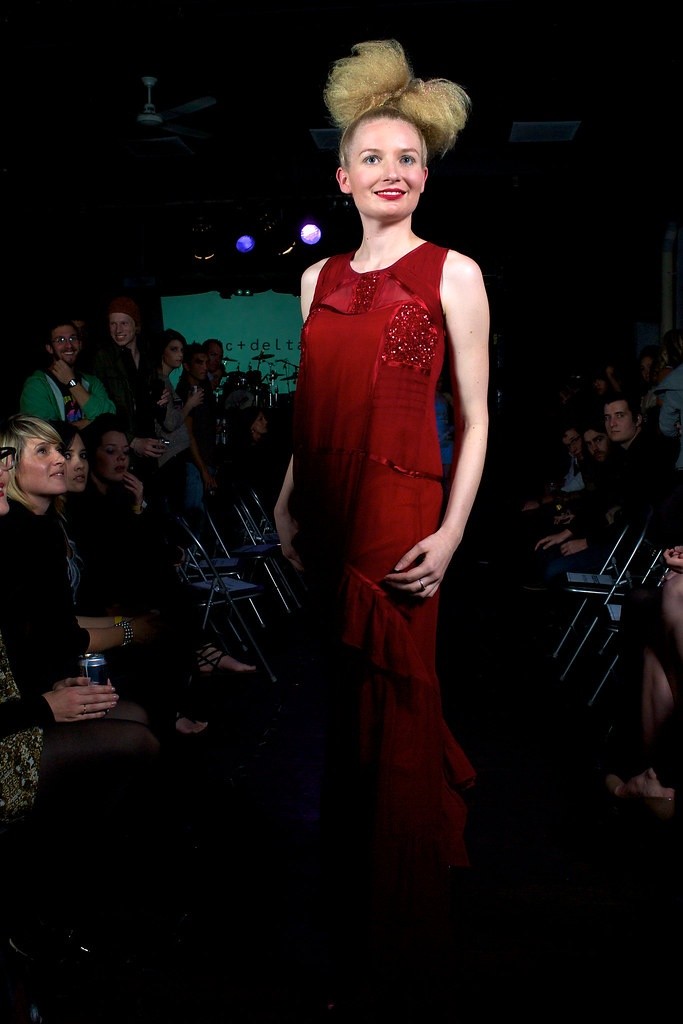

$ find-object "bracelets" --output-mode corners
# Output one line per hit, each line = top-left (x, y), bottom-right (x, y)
(115, 620), (134, 648)
(113, 616), (123, 626)
(132, 504), (144, 511)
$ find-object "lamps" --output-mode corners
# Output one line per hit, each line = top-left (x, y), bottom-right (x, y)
(192, 217), (214, 262)
(259, 213), (295, 255)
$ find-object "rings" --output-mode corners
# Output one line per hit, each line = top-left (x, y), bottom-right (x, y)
(83, 704), (87, 714)
(418, 579), (426, 591)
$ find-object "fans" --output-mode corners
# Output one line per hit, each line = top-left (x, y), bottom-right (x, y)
(137, 76), (217, 139)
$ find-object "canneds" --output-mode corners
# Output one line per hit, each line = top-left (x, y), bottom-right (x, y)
(78, 653), (107, 686)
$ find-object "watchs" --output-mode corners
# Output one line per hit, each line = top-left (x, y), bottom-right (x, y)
(65, 378), (79, 389)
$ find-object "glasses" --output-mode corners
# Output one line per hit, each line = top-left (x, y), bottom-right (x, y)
(51, 336), (78, 344)
(208, 351), (223, 361)
(564, 436), (580, 449)
(0, 446), (17, 471)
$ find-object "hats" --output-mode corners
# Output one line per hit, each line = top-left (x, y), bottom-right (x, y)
(109, 296), (141, 324)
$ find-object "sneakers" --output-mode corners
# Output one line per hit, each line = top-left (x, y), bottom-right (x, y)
(174, 711), (209, 735)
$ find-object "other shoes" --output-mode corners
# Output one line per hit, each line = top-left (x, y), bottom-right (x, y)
(605, 767), (676, 821)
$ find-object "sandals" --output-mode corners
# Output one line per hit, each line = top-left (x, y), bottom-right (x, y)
(192, 641), (258, 675)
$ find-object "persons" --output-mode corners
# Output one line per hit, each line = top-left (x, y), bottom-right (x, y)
(506, 328), (682, 822)
(1, 294), (316, 1024)
(266, 34), (493, 939)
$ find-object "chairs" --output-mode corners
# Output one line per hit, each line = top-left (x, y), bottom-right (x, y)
(175, 488), (310, 682)
(551, 507), (653, 680)
(588, 545), (671, 706)
